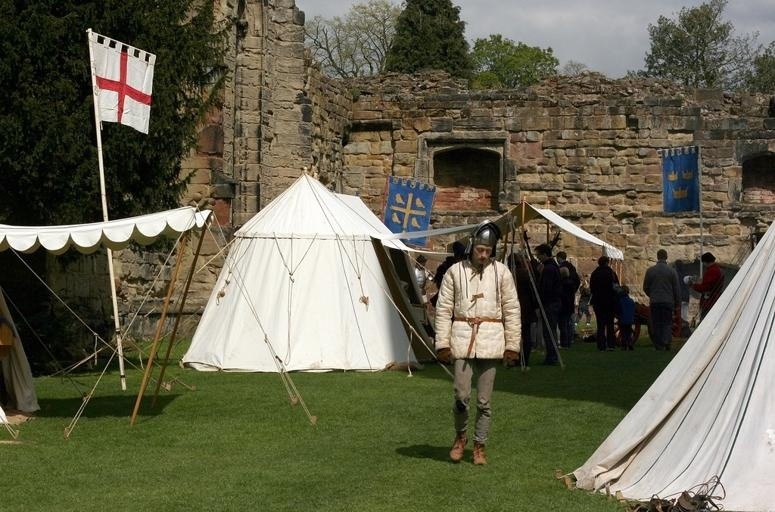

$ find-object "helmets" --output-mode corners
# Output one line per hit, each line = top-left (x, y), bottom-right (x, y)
(465, 219), (502, 259)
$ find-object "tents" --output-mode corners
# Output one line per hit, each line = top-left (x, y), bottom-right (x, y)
(180, 168), (435, 374)
(556, 220), (774, 512)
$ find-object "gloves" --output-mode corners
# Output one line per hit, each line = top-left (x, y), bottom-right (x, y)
(436, 348), (453, 366)
(503, 350), (520, 368)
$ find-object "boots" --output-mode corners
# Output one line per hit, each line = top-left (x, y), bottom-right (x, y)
(450, 430), (468, 461)
(472, 441), (488, 465)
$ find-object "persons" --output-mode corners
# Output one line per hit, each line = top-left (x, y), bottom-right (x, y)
(435, 225), (521, 465)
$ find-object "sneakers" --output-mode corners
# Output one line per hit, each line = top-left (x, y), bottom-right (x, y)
(597, 344), (672, 352)
(516, 356), (558, 367)
(557, 339), (574, 350)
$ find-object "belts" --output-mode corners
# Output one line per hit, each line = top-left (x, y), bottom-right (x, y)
(452, 316), (504, 356)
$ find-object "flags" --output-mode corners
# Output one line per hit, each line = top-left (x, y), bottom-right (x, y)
(92, 29), (157, 136)
